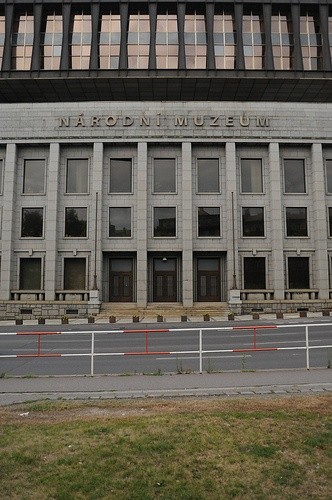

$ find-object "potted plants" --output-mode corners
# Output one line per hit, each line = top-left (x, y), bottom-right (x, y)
(61, 316), (68, 324)
(276, 313), (283, 319)
(228, 314), (234, 320)
(108, 315), (116, 322)
(203, 314), (210, 321)
(88, 316), (94, 323)
(156, 315), (164, 322)
(322, 310), (330, 316)
(38, 317), (45, 324)
(133, 316), (140, 322)
(16, 318), (23, 325)
(181, 316), (188, 321)
(299, 312), (307, 317)
(253, 313), (260, 319)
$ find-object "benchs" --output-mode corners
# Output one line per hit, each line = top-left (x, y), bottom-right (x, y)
(240, 289), (275, 300)
(11, 290), (45, 301)
(284, 288), (319, 300)
(55, 291), (90, 301)
